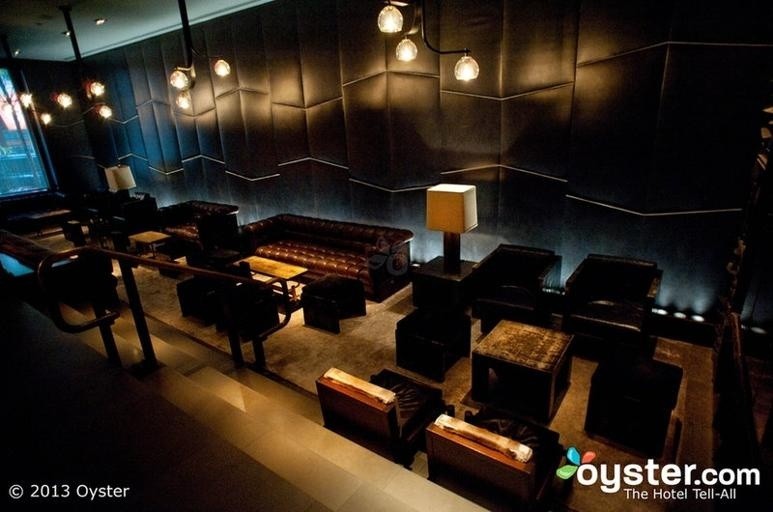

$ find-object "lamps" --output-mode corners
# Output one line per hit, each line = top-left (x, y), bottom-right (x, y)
(425, 183), (479, 272)
(57, 6), (112, 128)
(0, 28), (52, 126)
(104, 164), (136, 193)
(377, 0), (480, 81)
(166, 0), (231, 109)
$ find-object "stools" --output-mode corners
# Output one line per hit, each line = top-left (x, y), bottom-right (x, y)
(176, 267), (366, 340)
